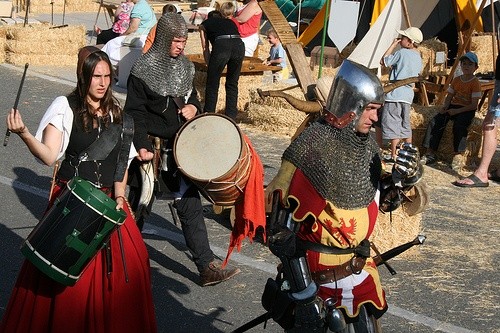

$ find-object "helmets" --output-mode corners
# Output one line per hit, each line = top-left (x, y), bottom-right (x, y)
(325, 58), (385, 129)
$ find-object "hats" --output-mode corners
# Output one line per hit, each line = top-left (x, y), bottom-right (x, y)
(458, 52), (478, 63)
(396, 27), (423, 44)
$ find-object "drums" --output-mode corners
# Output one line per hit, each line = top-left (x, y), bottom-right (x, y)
(19, 174), (127, 287)
(172, 111), (260, 215)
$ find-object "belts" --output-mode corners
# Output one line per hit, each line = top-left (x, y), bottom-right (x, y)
(215, 35), (240, 41)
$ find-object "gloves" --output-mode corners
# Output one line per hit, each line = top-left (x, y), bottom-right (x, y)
(390, 141), (425, 186)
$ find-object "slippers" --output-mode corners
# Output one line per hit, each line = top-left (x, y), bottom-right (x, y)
(453, 174), (489, 187)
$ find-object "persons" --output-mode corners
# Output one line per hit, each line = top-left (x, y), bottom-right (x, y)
(218, 0), (263, 58)
(100, 0), (158, 88)
(424, 52), (481, 166)
(259, 28), (288, 82)
(94, 2), (135, 44)
(260, 59), (425, 333)
(454, 28), (500, 187)
(0, 46), (158, 333)
(379, 26), (424, 164)
(198, 10), (245, 121)
(119, 11), (242, 286)
(140, 4), (178, 56)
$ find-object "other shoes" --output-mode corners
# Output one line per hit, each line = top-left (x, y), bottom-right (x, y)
(421, 154), (437, 164)
(200, 262), (241, 286)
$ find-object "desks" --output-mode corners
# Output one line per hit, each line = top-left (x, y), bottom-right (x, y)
(478, 77), (495, 107)
(184, 54), (282, 116)
